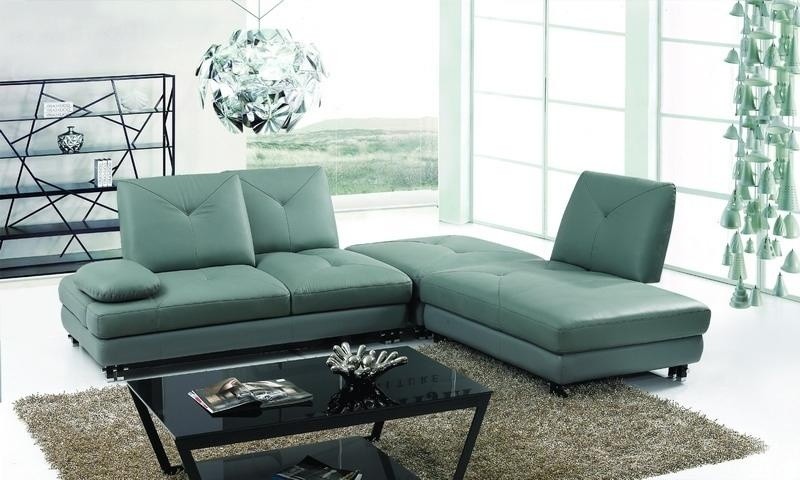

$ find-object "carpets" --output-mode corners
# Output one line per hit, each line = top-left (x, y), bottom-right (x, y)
(17, 341), (767, 479)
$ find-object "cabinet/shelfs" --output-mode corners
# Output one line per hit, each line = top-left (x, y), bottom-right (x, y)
(0, 73), (177, 280)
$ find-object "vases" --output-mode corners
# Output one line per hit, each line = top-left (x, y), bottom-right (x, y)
(57, 124), (84, 152)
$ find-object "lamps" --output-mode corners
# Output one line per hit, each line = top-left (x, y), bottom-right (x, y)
(192, 1), (326, 135)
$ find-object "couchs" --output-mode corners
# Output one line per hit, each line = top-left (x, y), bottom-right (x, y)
(343, 171), (712, 397)
(58, 166), (413, 382)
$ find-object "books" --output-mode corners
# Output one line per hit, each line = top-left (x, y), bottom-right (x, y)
(277, 454), (363, 479)
(94, 158), (112, 188)
(43, 101), (73, 118)
(188, 377), (314, 415)
